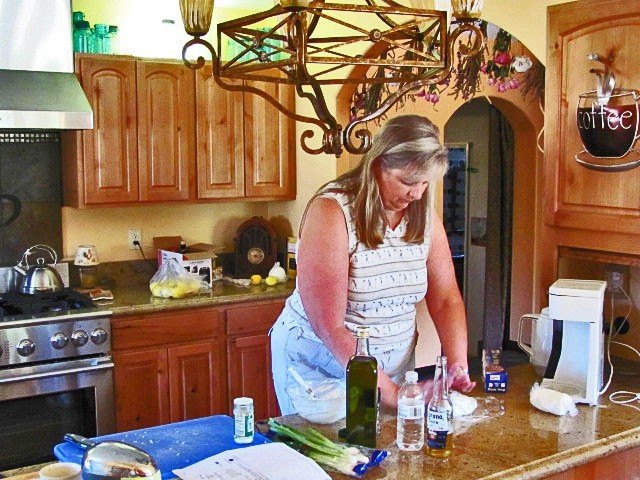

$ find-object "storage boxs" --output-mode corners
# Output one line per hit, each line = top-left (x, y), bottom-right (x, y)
(150, 236), (226, 291)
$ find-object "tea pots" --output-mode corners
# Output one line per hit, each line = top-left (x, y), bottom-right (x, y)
(13, 244), (66, 294)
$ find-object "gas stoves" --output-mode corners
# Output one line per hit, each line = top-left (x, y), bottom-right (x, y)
(0, 294), (116, 326)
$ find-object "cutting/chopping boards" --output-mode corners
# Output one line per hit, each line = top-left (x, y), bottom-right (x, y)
(53, 414), (274, 479)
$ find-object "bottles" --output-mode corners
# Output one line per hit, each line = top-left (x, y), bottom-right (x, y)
(426, 356), (453, 458)
(397, 371), (424, 451)
(74, 21), (90, 53)
(108, 25), (119, 55)
(233, 397), (254, 443)
(346, 326), (376, 447)
(88, 24), (111, 54)
(73, 12), (86, 32)
(226, 26), (283, 64)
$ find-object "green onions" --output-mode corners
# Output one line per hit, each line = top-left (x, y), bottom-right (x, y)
(267, 418), (371, 465)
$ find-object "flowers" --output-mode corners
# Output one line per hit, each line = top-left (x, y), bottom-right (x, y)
(479, 29), (531, 95)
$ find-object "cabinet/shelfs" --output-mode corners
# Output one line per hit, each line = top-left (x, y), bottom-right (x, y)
(225, 302), (286, 421)
(111, 297), (224, 432)
(197, 59), (297, 204)
(544, 3), (640, 235)
(61, 54), (196, 209)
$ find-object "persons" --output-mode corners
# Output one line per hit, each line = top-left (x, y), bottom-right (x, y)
(268, 114), (477, 417)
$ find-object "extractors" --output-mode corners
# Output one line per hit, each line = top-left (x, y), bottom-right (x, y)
(0, 72), (93, 130)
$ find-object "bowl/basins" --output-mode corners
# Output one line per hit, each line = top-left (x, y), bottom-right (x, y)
(39, 462), (82, 480)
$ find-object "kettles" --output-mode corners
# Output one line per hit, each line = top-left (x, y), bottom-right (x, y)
(517, 307), (554, 376)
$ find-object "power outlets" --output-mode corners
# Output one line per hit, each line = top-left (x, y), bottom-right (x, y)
(128, 230), (143, 251)
(602, 270), (626, 294)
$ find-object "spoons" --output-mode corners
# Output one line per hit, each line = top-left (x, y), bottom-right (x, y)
(64, 433), (161, 480)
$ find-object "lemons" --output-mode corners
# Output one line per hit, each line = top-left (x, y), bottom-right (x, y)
(150, 280), (201, 299)
(265, 276), (278, 286)
(250, 274), (262, 285)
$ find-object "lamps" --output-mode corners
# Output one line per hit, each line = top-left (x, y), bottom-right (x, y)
(177, 2), (487, 157)
